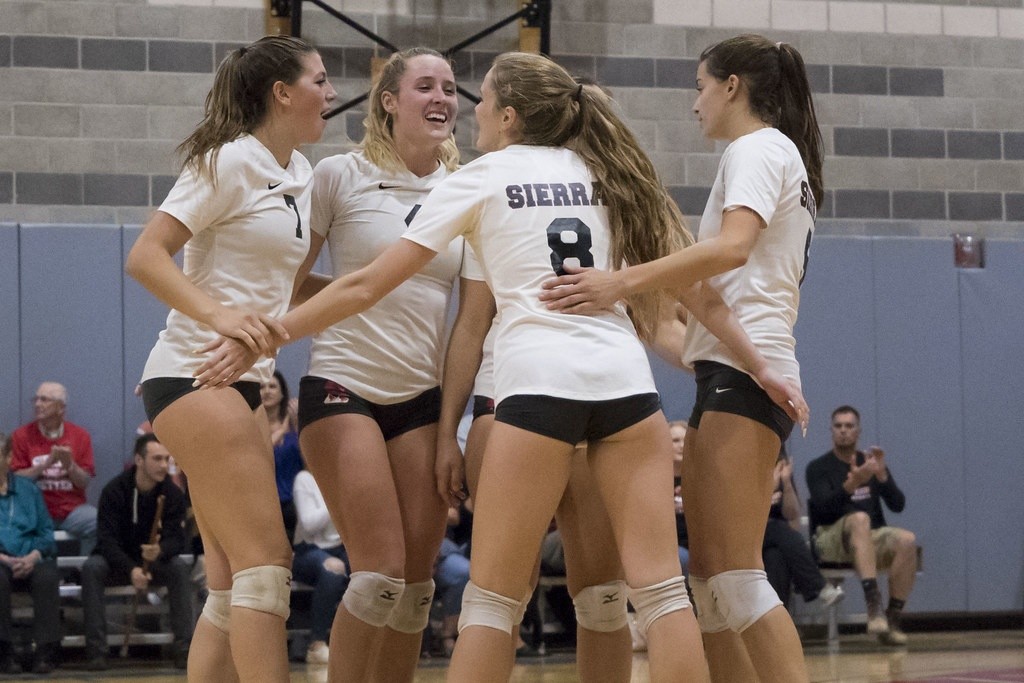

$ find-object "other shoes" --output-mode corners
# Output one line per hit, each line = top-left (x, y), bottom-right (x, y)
(86, 653), (109, 670)
(625, 611), (646, 651)
(33, 647), (54, 673)
(819, 582), (846, 608)
(516, 644), (529, 655)
(175, 650), (188, 668)
(436, 631), (456, 657)
(306, 642), (329, 664)
(0, 652), (22, 673)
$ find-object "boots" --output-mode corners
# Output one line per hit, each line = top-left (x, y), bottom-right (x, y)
(863, 590), (890, 633)
(880, 607), (908, 646)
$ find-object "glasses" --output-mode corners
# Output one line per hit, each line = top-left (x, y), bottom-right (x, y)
(33, 395), (58, 403)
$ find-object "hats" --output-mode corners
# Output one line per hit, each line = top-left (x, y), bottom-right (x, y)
(35, 381), (67, 404)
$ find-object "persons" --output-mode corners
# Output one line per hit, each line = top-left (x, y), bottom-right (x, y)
(0, 375), (919, 683)
(118, 35), (835, 683)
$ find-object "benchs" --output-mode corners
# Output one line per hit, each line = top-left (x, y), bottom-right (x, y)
(11, 516), (921, 659)
